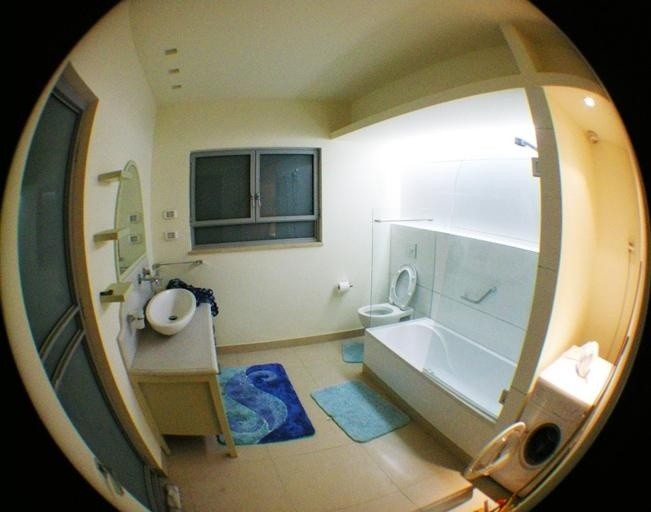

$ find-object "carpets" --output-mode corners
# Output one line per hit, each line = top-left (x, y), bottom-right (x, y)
(342, 341), (366, 363)
(310, 378), (411, 441)
(216, 362), (316, 446)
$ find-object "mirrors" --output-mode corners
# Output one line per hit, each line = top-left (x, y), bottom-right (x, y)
(115, 161), (148, 283)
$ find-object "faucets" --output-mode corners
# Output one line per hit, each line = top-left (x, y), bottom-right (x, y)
(137, 274), (161, 285)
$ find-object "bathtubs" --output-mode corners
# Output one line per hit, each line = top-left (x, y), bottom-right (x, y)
(362, 317), (518, 459)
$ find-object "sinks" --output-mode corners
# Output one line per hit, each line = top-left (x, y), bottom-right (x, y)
(145, 287), (198, 336)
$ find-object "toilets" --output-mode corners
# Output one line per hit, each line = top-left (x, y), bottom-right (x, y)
(358, 262), (417, 327)
